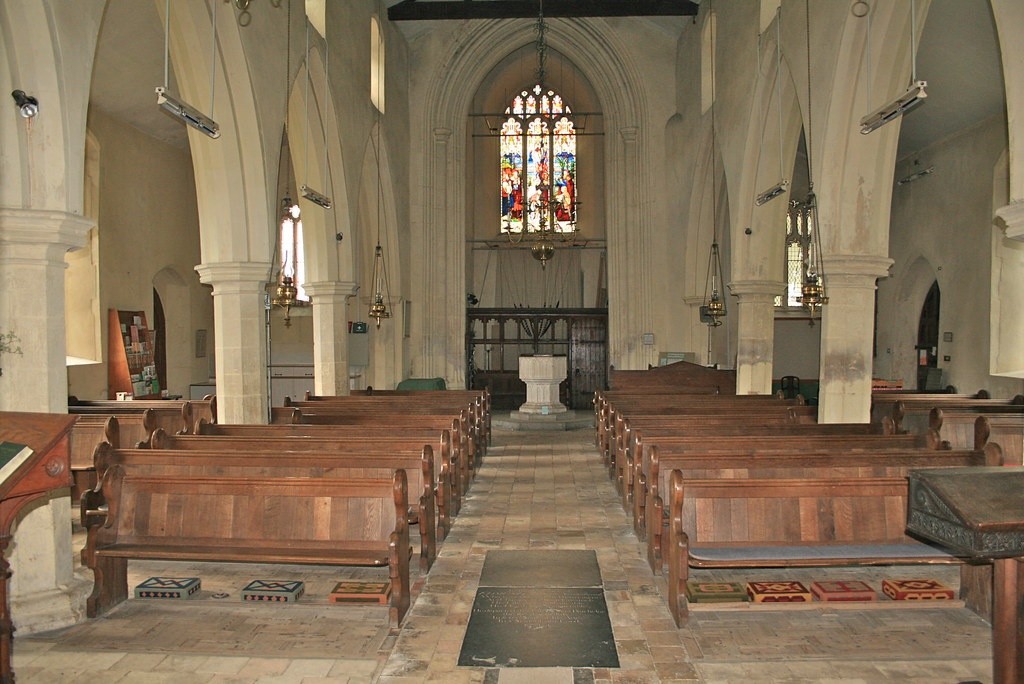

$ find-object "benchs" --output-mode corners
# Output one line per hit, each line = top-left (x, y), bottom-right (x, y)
(70, 388), (491, 626)
(593, 360), (1024, 630)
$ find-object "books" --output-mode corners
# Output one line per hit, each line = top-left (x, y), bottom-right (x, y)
(121, 316), (159, 396)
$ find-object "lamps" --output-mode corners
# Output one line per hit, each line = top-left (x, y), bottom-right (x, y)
(368, 1), (393, 330)
(155, 0), (221, 140)
(298, 15), (331, 210)
(897, 167), (934, 186)
(796, 1), (828, 327)
(859, 0), (928, 135)
(700, 0), (728, 328)
(11, 89), (39, 118)
(271, 1), (303, 326)
(755, 6), (789, 206)
(503, 195), (583, 271)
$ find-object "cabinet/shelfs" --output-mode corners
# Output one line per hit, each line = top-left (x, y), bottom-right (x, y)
(107, 308), (162, 400)
